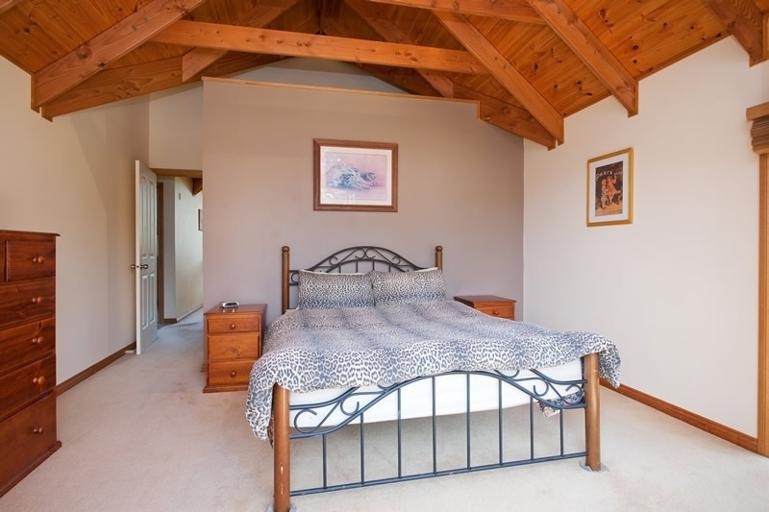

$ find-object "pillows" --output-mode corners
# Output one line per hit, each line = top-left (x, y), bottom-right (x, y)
(296, 267), (447, 311)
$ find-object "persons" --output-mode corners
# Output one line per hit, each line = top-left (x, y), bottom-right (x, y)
(606, 175), (621, 205)
(601, 179), (608, 209)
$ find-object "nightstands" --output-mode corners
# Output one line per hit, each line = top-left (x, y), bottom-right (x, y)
(454, 294), (516, 321)
(202, 303), (268, 393)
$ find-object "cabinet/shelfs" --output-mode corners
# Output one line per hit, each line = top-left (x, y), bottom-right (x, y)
(0, 229), (62, 498)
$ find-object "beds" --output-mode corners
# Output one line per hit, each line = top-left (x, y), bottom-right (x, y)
(244, 246), (622, 512)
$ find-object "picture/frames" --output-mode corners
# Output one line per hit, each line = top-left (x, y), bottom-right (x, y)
(198, 208), (203, 230)
(312, 138), (400, 212)
(586, 147), (635, 226)
